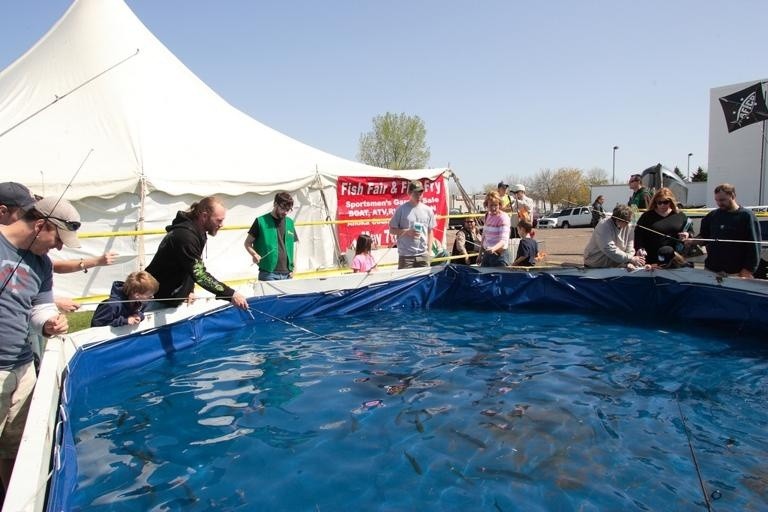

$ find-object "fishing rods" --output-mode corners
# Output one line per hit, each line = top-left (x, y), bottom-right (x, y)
(251, 308), (335, 340)
(423, 223), (480, 248)
(675, 392), (721, 511)
(78, 294), (240, 314)
(681, 237), (768, 245)
(561, 197), (677, 239)
(364, 228), (407, 275)
(612, 266), (649, 281)
(1, 146), (97, 296)
(1, 47), (140, 135)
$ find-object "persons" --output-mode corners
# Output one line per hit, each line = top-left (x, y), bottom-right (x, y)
(389, 181), (435, 270)
(89, 270), (159, 328)
(582, 174), (761, 282)
(0, 181), (120, 275)
(141, 196), (250, 311)
(0, 195), (68, 458)
(245, 193), (298, 280)
(450, 182), (539, 267)
(352, 235), (376, 274)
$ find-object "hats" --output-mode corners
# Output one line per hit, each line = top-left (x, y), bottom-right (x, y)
(408, 180), (424, 193)
(0, 181), (35, 213)
(497, 180), (508, 188)
(509, 183), (526, 194)
(33, 197), (84, 249)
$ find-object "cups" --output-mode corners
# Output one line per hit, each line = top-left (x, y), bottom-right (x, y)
(412, 223), (422, 240)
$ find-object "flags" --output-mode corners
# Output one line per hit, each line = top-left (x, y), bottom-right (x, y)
(718, 82), (768, 134)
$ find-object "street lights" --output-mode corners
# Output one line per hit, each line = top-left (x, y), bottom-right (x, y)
(687, 153), (693, 182)
(613, 146), (620, 185)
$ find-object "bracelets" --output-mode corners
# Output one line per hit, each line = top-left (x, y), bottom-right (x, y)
(80, 258), (88, 273)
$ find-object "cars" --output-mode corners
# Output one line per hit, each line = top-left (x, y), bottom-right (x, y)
(447, 206), (593, 229)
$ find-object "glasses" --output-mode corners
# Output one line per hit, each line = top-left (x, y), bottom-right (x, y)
(39, 215), (81, 231)
